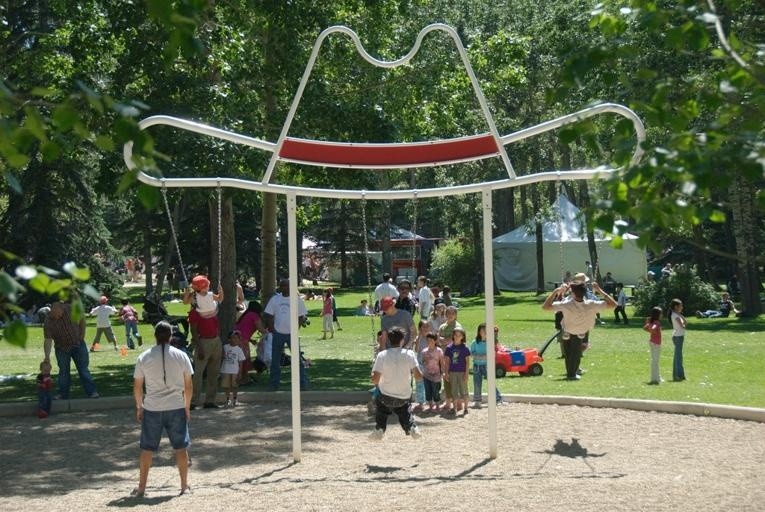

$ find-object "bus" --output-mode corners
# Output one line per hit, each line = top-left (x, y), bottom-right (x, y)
(329, 237), (445, 285)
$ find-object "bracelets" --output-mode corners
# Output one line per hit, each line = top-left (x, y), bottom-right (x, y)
(135, 403), (145, 410)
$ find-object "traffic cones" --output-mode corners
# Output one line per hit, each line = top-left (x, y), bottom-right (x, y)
(119, 342), (129, 357)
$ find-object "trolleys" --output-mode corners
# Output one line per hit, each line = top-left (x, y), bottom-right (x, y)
(497, 330), (562, 377)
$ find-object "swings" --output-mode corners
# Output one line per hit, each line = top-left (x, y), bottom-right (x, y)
(161, 187), (223, 318)
(555, 179), (611, 343)
(362, 197), (420, 413)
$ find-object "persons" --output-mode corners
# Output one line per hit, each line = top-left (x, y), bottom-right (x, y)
(37, 362), (56, 418)
(119, 300), (142, 349)
(126, 255), (142, 283)
(130, 320), (195, 499)
(321, 288), (343, 338)
(358, 272), (501, 439)
(43, 302), (100, 399)
(183, 275), (308, 408)
(542, 261), (742, 385)
(90, 296), (118, 352)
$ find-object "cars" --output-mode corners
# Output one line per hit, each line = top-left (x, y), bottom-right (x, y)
(648, 249), (731, 285)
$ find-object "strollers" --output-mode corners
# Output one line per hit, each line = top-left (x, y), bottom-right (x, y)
(250, 331), (311, 383)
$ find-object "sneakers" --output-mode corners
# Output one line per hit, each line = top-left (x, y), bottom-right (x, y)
(368, 428), (384, 440)
(410, 426), (421, 438)
(138, 336), (142, 345)
(203, 403), (219, 409)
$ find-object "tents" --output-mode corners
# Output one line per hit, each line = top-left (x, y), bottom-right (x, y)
(493, 193), (648, 292)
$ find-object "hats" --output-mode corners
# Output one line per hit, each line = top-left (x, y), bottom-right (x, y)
(191, 276), (209, 290)
(228, 329), (241, 337)
(100, 296), (108, 304)
(574, 273), (590, 284)
(379, 297), (393, 311)
